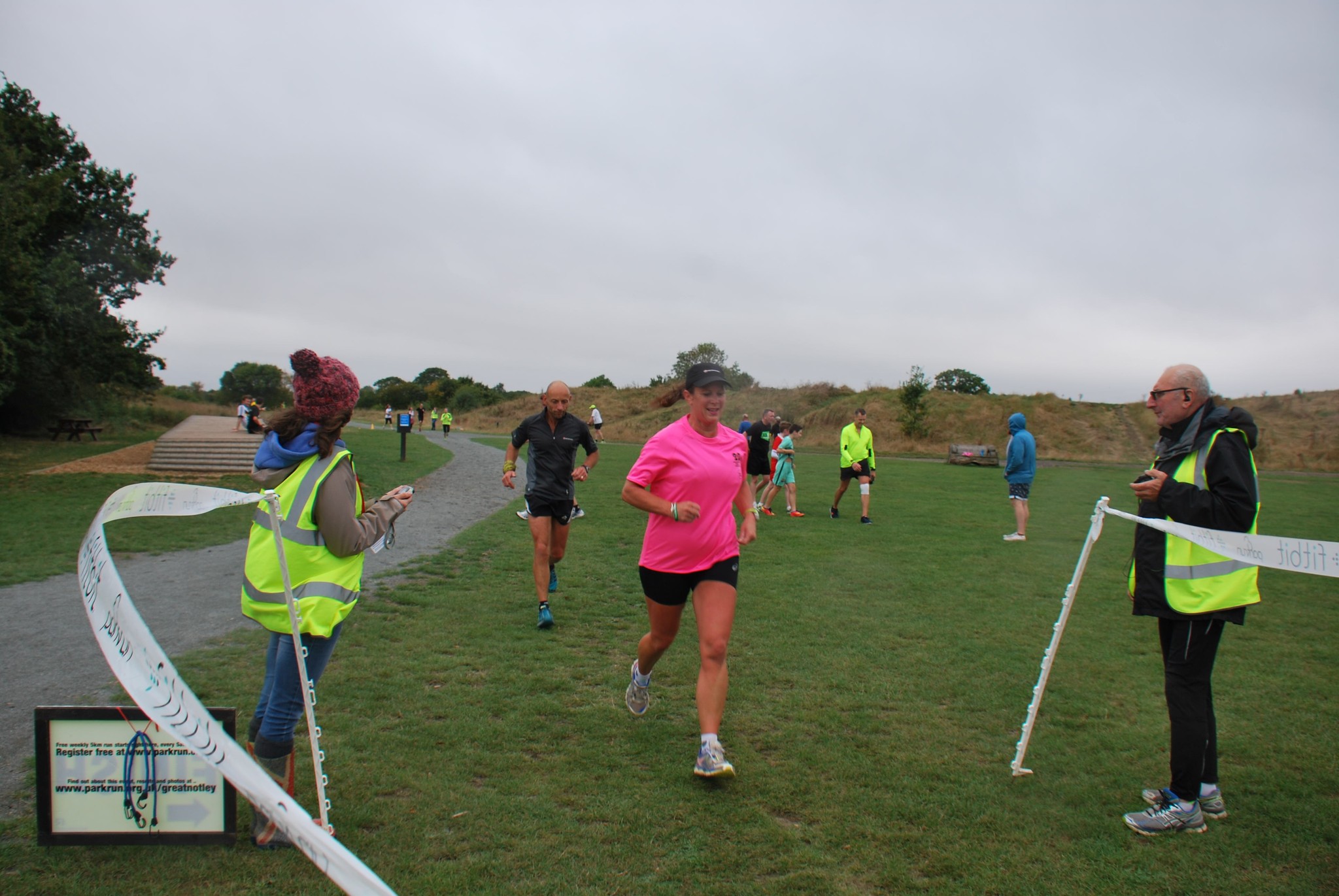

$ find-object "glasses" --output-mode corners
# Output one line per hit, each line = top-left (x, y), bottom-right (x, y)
(1150, 387), (1189, 400)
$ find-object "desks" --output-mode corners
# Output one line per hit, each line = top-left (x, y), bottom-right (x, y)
(51, 418), (99, 442)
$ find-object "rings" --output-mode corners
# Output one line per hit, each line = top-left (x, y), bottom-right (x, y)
(581, 476), (584, 479)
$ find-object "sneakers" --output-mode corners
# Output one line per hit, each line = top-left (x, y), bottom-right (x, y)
(516, 510), (529, 520)
(537, 605), (554, 628)
(570, 508), (585, 519)
(790, 511), (804, 517)
(757, 504), (764, 510)
(626, 659), (651, 715)
(694, 740), (735, 778)
(762, 506), (775, 515)
(548, 571), (557, 592)
(753, 504), (757, 510)
(1142, 787), (1228, 820)
(830, 505), (838, 519)
(861, 516), (872, 524)
(1003, 532), (1026, 541)
(786, 509), (801, 514)
(1120, 788), (1207, 836)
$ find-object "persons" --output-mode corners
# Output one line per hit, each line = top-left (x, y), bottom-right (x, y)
(501, 381), (599, 626)
(1122, 363), (1261, 836)
(441, 408), (453, 440)
(736, 409), (805, 517)
(407, 407), (415, 429)
(622, 363), (758, 778)
(281, 401), (286, 408)
(415, 403), (425, 431)
(588, 405), (604, 444)
(384, 404), (393, 429)
(1002, 413), (1036, 541)
(232, 395), (267, 435)
(829, 408), (876, 525)
(242, 349), (414, 847)
(431, 407), (438, 430)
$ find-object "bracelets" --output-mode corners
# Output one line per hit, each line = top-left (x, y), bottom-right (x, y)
(871, 469), (875, 471)
(670, 502), (678, 521)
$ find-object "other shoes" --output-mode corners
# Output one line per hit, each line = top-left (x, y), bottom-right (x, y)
(594, 441), (596, 443)
(601, 441), (604, 443)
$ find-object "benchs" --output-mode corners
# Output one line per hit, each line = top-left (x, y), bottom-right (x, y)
(48, 426), (104, 433)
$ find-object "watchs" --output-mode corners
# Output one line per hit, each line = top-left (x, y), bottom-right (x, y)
(744, 509), (759, 521)
(581, 465), (589, 473)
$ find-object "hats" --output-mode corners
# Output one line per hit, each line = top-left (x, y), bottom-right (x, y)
(589, 404), (596, 409)
(290, 349), (360, 419)
(685, 362), (733, 389)
(776, 416), (781, 422)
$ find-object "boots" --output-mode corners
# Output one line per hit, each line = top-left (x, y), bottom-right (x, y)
(247, 741), (322, 829)
(254, 748), (336, 845)
